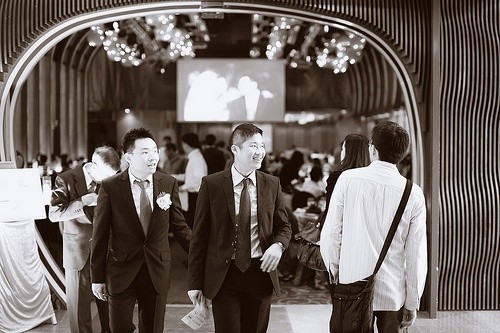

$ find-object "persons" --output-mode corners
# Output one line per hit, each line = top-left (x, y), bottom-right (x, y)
(184, 69), (282, 121)
(187, 123), (292, 333)
(49, 146), (121, 333)
(90, 127), (192, 333)
(15, 133), (412, 305)
(320, 121), (428, 333)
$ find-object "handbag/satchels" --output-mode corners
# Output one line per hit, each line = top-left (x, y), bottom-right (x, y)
(330, 277), (375, 333)
(294, 221), (327, 270)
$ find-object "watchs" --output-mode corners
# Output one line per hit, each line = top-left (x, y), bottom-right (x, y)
(277, 242), (285, 251)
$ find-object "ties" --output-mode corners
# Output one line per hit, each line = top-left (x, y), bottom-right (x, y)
(235, 178), (251, 272)
(137, 182), (152, 236)
(89, 181), (96, 193)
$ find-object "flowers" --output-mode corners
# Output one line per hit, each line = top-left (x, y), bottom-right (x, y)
(155, 192), (173, 211)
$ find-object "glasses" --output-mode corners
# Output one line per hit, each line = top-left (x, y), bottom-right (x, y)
(368, 140), (373, 145)
(142, 150), (160, 156)
